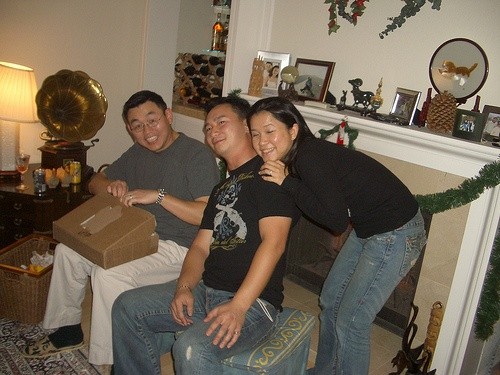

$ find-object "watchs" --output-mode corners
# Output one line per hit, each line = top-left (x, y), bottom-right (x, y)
(156, 188), (168, 205)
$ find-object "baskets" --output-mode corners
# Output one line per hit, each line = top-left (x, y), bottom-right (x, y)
(0, 234), (60, 324)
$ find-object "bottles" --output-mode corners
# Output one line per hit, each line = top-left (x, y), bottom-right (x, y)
(220, 14), (230, 52)
(211, 12), (223, 51)
(174, 52), (223, 110)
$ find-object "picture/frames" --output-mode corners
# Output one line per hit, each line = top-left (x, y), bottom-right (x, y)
(481, 105), (500, 143)
(391, 87), (422, 126)
(289, 58), (336, 103)
(452, 109), (488, 142)
(429, 38), (490, 104)
(257, 50), (291, 97)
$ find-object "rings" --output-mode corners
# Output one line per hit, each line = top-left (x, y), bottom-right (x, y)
(130, 194), (132, 198)
(235, 332), (240, 335)
(270, 170), (272, 175)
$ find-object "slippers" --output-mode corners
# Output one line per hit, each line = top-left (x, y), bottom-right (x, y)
(20, 335), (87, 358)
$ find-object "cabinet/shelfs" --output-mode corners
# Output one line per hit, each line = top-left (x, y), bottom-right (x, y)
(0, 162), (95, 248)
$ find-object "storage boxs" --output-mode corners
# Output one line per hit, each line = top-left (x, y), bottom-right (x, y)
(53, 191), (160, 270)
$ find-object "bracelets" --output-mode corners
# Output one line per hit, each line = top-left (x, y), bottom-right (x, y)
(177, 285), (192, 291)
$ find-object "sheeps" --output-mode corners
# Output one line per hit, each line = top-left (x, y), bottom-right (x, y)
(349, 78), (374, 110)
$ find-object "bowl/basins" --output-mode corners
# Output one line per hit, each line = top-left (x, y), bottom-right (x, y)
(59, 175), (73, 187)
(46, 176), (58, 188)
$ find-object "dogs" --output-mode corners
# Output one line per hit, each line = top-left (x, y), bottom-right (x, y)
(439, 61), (478, 86)
(339, 90), (347, 106)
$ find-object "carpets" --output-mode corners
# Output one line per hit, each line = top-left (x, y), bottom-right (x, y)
(0, 314), (106, 375)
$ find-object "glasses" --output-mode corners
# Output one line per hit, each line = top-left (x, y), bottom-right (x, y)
(127, 111), (165, 134)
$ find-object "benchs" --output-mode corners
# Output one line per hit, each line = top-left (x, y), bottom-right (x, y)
(80, 269), (319, 375)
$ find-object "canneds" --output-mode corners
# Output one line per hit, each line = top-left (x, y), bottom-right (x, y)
(69, 162), (81, 184)
(33, 168), (46, 194)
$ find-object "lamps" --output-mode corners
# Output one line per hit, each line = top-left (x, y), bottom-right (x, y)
(0, 62), (42, 187)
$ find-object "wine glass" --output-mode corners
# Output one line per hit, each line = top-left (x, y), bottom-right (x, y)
(15, 154), (30, 190)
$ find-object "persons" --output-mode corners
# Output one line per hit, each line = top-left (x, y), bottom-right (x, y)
(112, 95), (301, 374)
(246, 96), (429, 375)
(20, 90), (221, 365)
(260, 60), (281, 90)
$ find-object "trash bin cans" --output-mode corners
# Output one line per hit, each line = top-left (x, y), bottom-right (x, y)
(0, 233), (60, 323)
